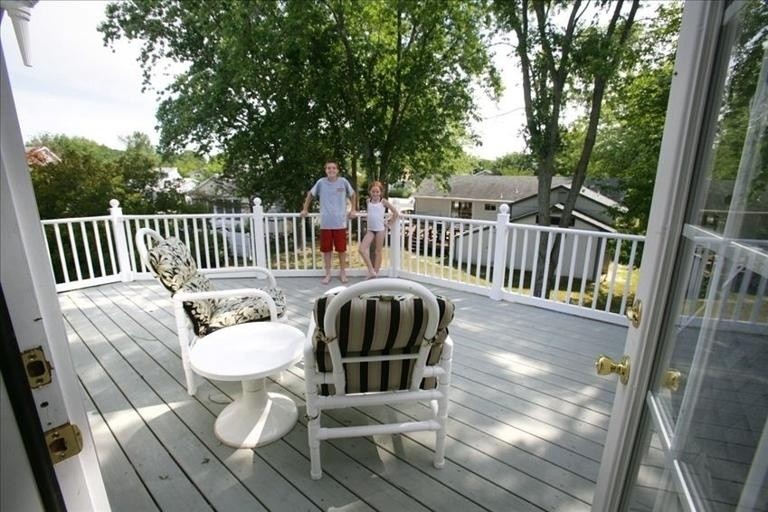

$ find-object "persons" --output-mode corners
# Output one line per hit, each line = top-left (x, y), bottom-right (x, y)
(357, 180), (400, 281)
(300, 158), (358, 284)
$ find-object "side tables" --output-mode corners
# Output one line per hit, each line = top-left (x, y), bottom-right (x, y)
(190, 322), (306, 448)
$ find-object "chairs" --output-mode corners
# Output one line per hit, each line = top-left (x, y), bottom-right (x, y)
(306, 278), (453, 480)
(135, 227), (288, 396)
